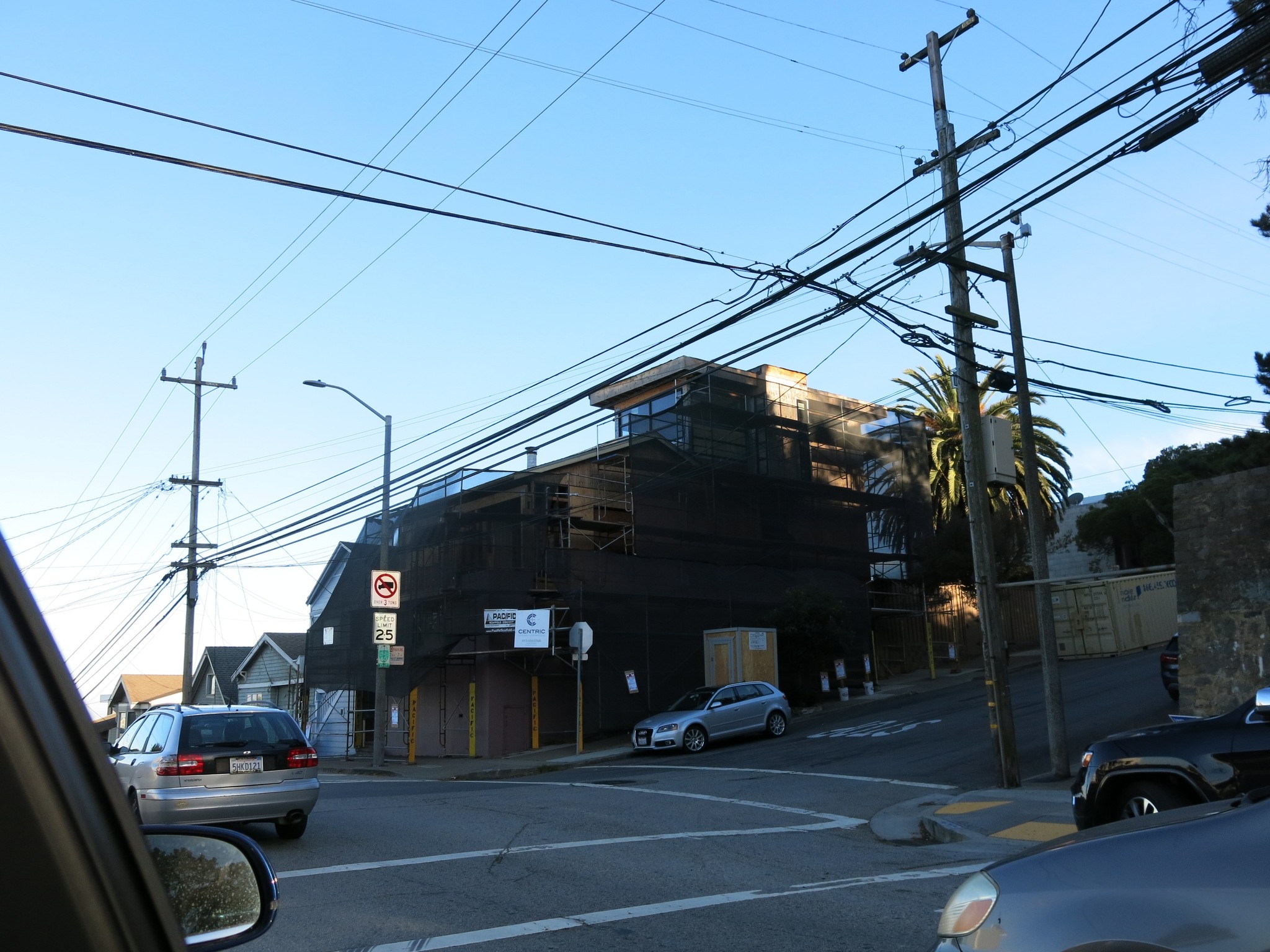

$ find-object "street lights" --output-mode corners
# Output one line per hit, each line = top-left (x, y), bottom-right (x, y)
(302, 379), (391, 766)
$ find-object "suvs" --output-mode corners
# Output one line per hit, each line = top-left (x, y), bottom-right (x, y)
(1160, 631), (1180, 702)
(98, 703), (321, 842)
(927, 686), (1269, 952)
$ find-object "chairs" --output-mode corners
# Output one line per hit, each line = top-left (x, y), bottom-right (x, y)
(219, 721), (268, 746)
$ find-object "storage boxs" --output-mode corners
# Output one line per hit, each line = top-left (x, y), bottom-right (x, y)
(1049, 570), (1177, 661)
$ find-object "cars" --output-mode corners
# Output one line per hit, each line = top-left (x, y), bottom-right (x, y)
(630, 680), (792, 753)
